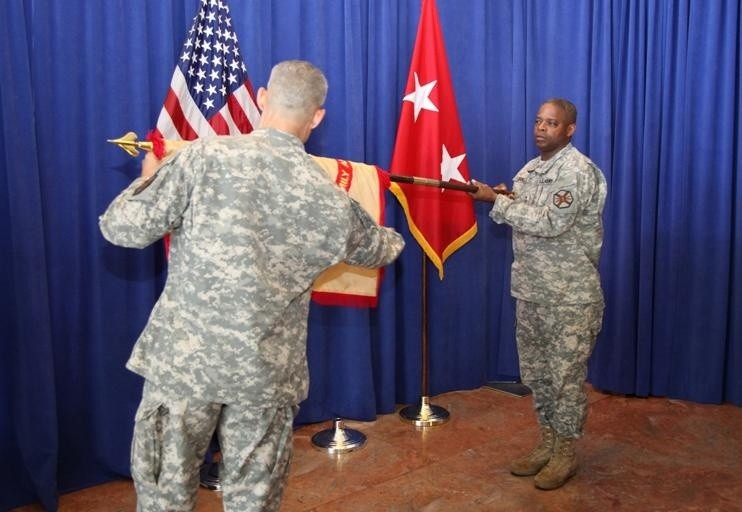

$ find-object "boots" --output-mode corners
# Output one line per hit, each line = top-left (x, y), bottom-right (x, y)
(511, 425), (553, 476)
(535, 437), (577, 489)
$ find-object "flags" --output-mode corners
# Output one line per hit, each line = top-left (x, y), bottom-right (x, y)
(387, 0), (481, 281)
(146, 131), (392, 311)
(144, 2), (265, 140)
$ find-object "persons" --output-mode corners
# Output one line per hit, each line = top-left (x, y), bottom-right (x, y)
(467, 98), (610, 489)
(98, 60), (405, 512)
